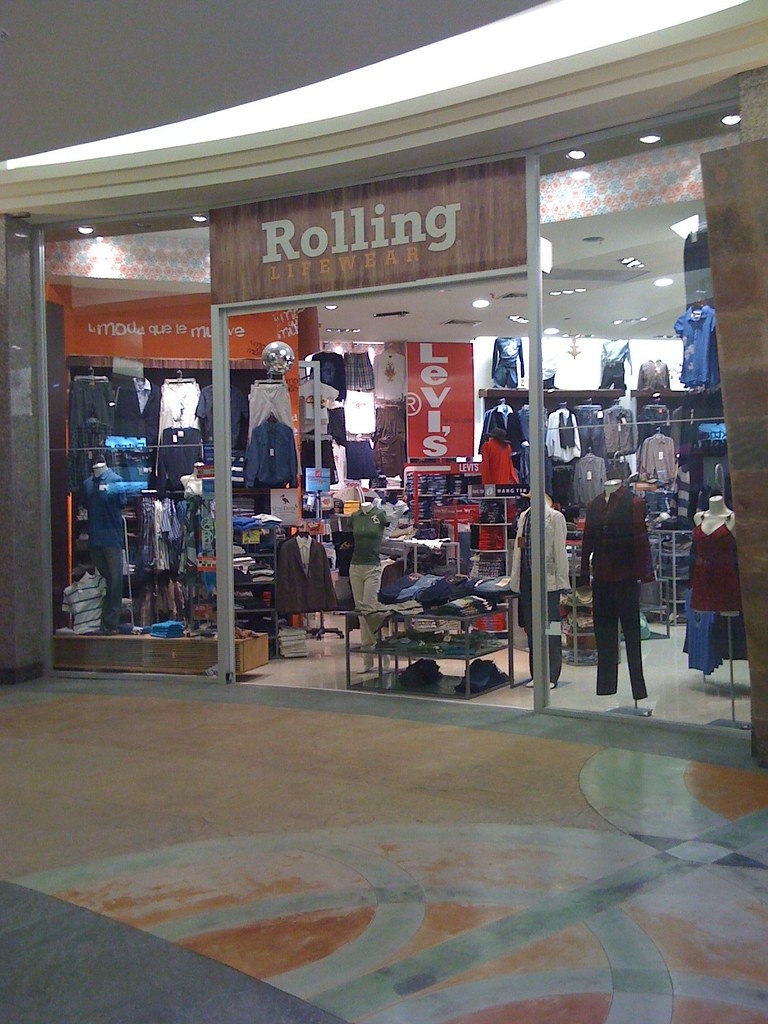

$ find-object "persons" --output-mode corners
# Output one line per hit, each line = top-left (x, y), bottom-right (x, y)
(690, 495), (742, 617)
(510, 500), (571, 689)
(346, 502), (390, 673)
(637, 357), (670, 389)
(598, 339), (633, 390)
(180, 462), (216, 516)
(80, 462), (127, 629)
(492, 337), (524, 388)
(581, 480), (656, 700)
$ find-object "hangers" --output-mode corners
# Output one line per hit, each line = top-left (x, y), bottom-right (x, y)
(74, 368), (109, 383)
(323, 341), (397, 354)
(266, 413), (277, 423)
(653, 427), (663, 437)
(692, 290), (708, 315)
(254, 372), (286, 387)
(499, 398), (507, 408)
(165, 370), (196, 383)
(375, 399), (401, 410)
(644, 393), (667, 410)
(573, 398), (602, 411)
(586, 446), (594, 458)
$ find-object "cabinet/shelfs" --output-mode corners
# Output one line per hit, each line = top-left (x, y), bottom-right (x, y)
(659, 529), (692, 626)
(561, 544), (623, 667)
(338, 607), (510, 700)
(188, 528), (280, 660)
(467, 495), (521, 635)
